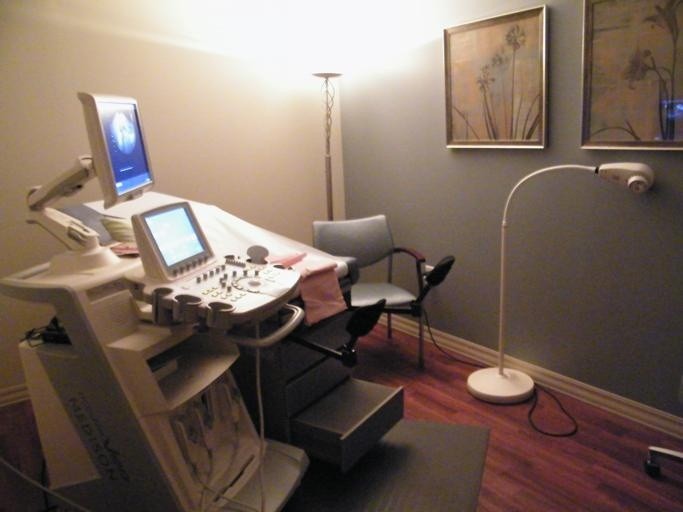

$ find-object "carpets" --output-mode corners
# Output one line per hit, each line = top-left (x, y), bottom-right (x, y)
(281, 419), (490, 512)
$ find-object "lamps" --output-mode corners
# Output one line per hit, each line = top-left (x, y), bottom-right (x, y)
(467, 162), (655, 406)
(313, 72), (342, 220)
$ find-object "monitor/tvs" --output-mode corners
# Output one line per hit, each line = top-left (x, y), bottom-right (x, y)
(76, 90), (156, 209)
(131, 201), (216, 281)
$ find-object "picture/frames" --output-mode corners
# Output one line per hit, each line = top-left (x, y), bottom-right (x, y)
(579, 0), (683, 153)
(443, 4), (547, 149)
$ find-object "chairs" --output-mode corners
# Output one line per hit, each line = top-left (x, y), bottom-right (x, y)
(312, 215), (426, 369)
